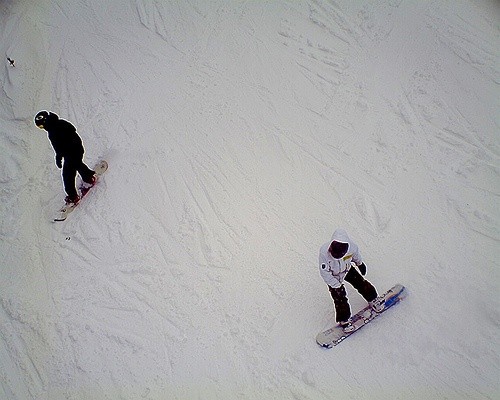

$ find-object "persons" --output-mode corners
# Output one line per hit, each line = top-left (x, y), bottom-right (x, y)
(34, 110), (95, 203)
(319, 229), (384, 333)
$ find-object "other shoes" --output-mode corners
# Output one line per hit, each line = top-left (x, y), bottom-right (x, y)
(340, 318), (354, 333)
(82, 174), (97, 188)
(368, 298), (384, 313)
(63, 195), (80, 208)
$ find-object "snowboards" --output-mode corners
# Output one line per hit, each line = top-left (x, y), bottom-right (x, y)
(315, 284), (409, 349)
(53, 160), (108, 221)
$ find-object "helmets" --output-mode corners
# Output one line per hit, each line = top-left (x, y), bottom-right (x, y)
(34, 112), (48, 124)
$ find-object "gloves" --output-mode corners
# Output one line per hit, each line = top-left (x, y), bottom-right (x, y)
(358, 263), (366, 276)
(336, 284), (347, 297)
(55, 156), (62, 168)
